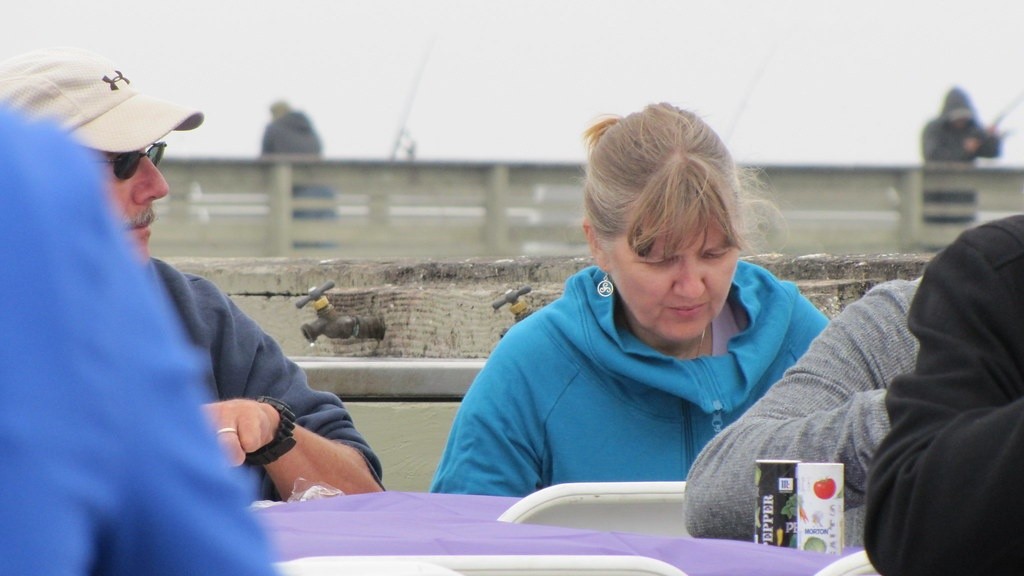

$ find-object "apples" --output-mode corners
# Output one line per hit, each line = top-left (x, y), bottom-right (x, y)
(813, 479), (836, 499)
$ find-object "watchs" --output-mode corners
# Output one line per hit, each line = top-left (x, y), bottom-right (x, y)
(242, 397), (298, 466)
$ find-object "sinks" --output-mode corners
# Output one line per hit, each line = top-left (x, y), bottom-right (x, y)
(286, 354), (485, 399)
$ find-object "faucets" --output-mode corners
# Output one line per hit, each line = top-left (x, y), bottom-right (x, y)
(491, 285), (540, 337)
(295, 281), (386, 342)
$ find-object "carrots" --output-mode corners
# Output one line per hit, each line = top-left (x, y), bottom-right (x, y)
(800, 507), (808, 522)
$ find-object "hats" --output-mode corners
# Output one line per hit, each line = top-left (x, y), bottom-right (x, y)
(0, 44), (205, 153)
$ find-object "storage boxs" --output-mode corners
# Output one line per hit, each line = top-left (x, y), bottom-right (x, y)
(755, 458), (846, 555)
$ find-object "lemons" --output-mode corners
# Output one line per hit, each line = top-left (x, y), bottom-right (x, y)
(803, 537), (826, 553)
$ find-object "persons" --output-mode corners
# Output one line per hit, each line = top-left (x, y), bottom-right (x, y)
(253, 98), (319, 247)
(0, 100), (281, 576)
(0, 45), (388, 502)
(862, 213), (1024, 576)
(922, 82), (1003, 229)
(686, 281), (922, 543)
(433, 102), (831, 499)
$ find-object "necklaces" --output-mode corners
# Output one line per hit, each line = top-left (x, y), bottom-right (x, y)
(697, 328), (707, 356)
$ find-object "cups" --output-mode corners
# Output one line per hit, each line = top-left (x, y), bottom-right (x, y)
(796, 462), (845, 555)
(754, 459), (800, 548)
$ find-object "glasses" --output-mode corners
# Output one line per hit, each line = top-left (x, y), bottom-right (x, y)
(92, 140), (167, 181)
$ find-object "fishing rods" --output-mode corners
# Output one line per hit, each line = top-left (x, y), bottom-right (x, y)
(389, 42), (438, 161)
(962, 89), (1024, 165)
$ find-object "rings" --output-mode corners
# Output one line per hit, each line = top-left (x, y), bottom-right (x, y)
(215, 428), (237, 436)
